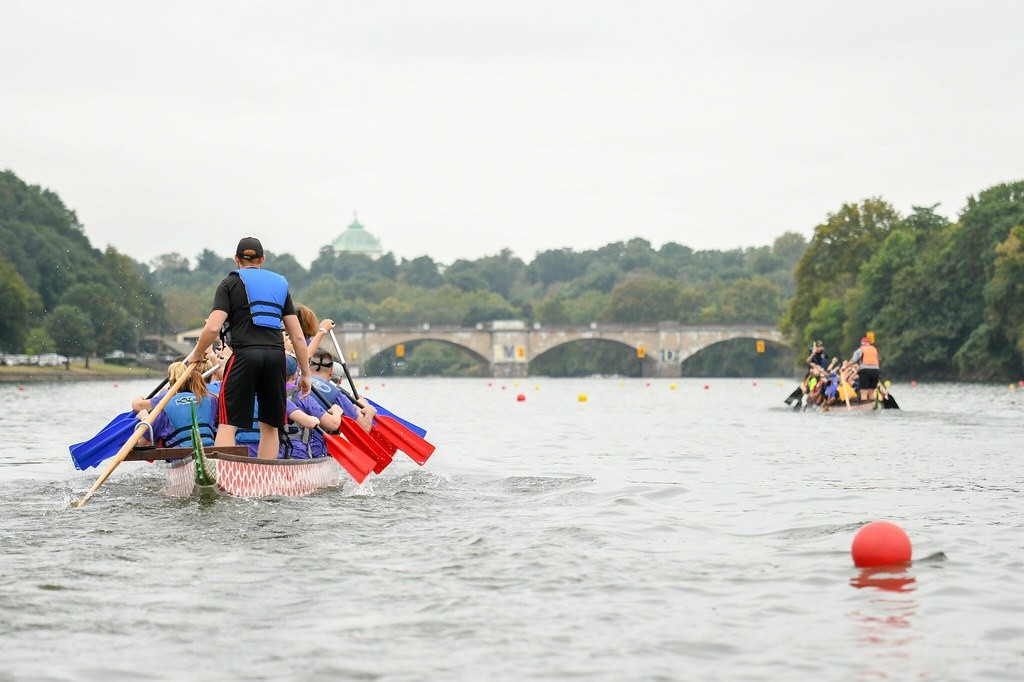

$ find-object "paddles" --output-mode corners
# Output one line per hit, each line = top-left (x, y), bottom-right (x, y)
(78, 347), (212, 508)
(67, 324), (224, 472)
(310, 384), (394, 477)
(840, 372), (852, 411)
(783, 370), (834, 413)
(879, 377), (900, 409)
(320, 320), (437, 468)
(313, 424), (378, 485)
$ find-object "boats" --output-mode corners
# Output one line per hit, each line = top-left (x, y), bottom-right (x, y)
(158, 442), (339, 504)
(807, 382), (879, 414)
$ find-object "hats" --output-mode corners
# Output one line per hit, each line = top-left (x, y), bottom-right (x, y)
(285, 354), (298, 376)
(237, 237), (263, 260)
(861, 337), (870, 342)
(815, 339), (823, 346)
(331, 361), (344, 380)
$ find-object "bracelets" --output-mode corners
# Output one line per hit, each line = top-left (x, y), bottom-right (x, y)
(319, 328), (327, 333)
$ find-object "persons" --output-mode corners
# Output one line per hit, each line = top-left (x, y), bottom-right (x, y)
(132, 303), (378, 460)
(800, 337), (880, 405)
(188, 238), (311, 460)
